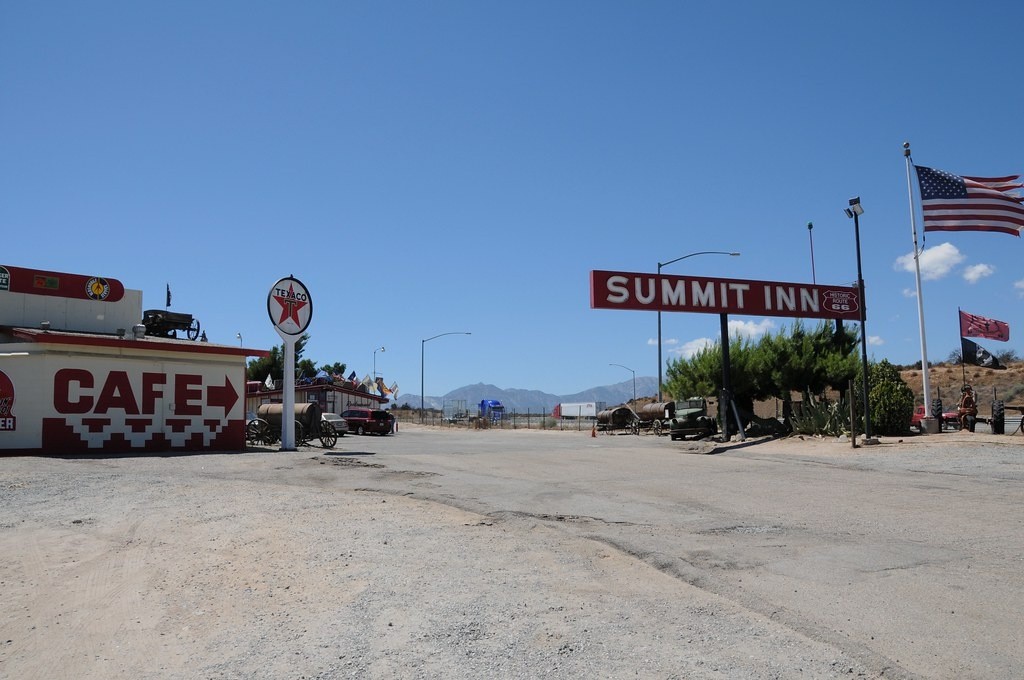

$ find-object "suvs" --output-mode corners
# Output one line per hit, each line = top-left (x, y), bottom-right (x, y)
(340, 408), (390, 436)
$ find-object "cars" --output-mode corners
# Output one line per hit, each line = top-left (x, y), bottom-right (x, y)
(321, 412), (349, 436)
(669, 397), (717, 440)
(246, 411), (259, 431)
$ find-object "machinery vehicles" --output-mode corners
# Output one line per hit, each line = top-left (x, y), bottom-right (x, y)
(933, 386), (1005, 435)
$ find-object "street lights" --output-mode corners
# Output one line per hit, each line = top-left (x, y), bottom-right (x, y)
(610, 363), (636, 413)
(843, 197), (873, 439)
(237, 332), (242, 346)
(657, 251), (741, 404)
(373, 346), (384, 384)
(422, 332), (471, 422)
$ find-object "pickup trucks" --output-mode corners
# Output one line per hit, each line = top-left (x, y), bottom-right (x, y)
(911, 406), (961, 432)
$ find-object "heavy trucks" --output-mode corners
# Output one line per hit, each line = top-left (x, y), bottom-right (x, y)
(451, 400), (510, 426)
(550, 402), (606, 420)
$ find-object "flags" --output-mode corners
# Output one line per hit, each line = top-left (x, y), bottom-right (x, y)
(265, 374), (275, 390)
(915, 166), (1024, 237)
(962, 338), (1007, 370)
(300, 369), (399, 400)
(960, 311), (1009, 342)
